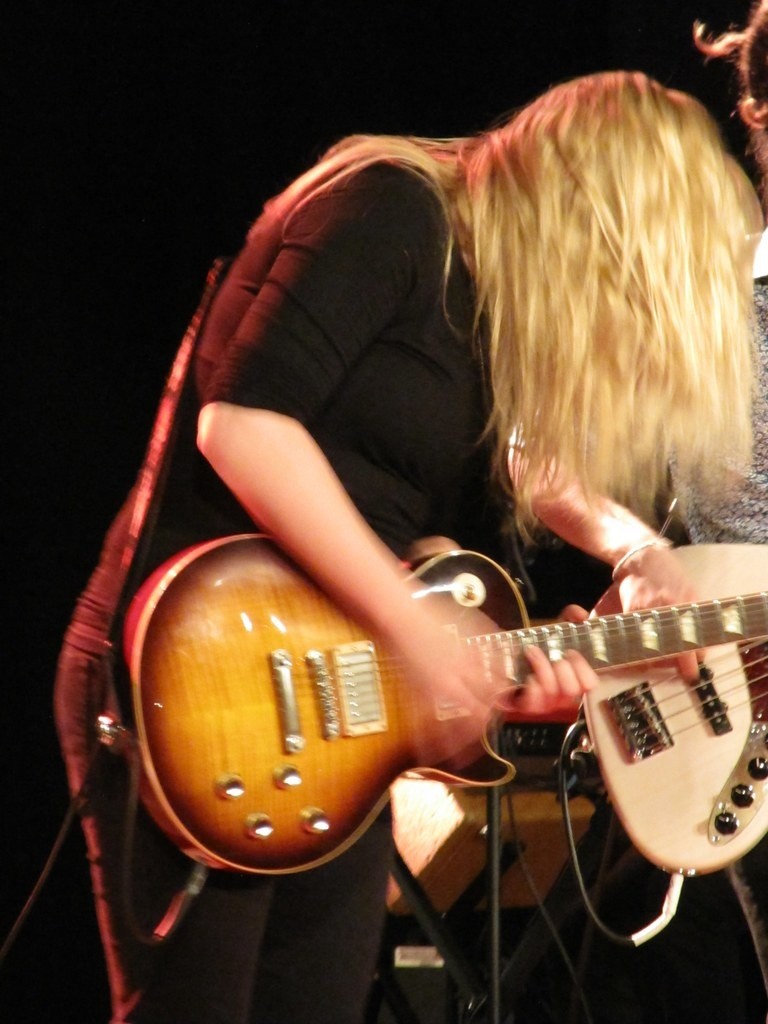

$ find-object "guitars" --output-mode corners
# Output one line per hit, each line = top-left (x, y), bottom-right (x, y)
(577, 541), (768, 878)
(117, 531), (768, 875)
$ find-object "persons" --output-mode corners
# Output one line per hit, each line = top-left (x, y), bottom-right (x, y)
(504, 0), (768, 1024)
(54, 72), (768, 1024)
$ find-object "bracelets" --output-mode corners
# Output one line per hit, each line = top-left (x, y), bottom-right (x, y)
(613, 541), (669, 580)
